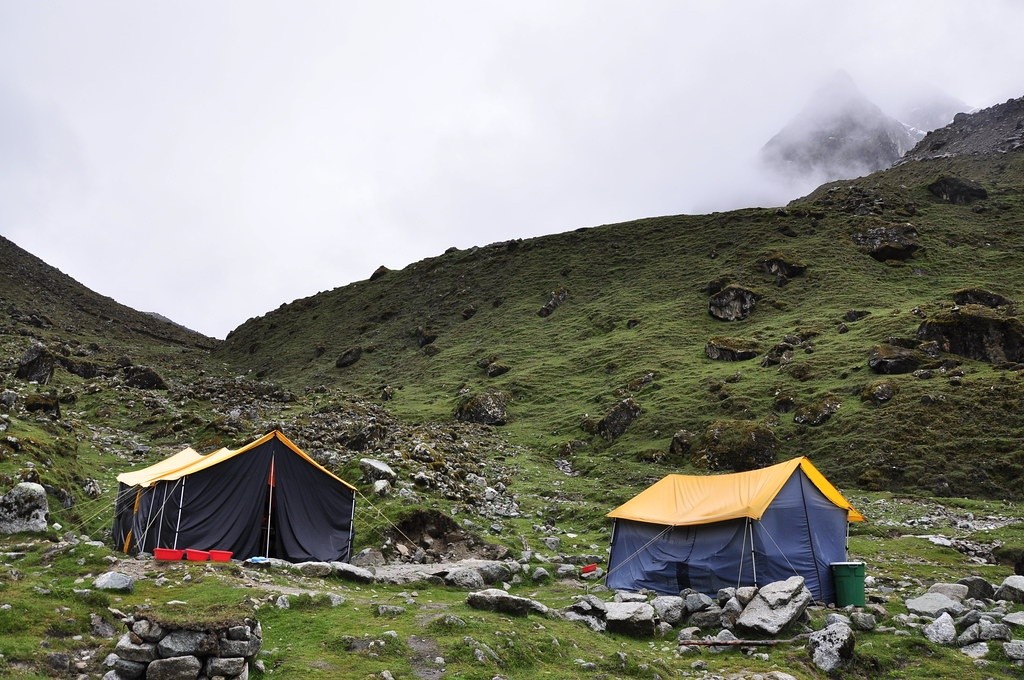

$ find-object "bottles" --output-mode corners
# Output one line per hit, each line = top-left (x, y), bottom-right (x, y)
(252, 557), (267, 562)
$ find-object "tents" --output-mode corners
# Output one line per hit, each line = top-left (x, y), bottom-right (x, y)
(113, 427), (358, 563)
(602, 454), (868, 605)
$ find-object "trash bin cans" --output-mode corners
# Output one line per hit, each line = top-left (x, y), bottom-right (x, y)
(829, 561), (866, 607)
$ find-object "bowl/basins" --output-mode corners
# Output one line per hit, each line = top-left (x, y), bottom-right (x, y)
(184, 548), (209, 560)
(209, 550), (233, 562)
(154, 547), (185, 561)
(582, 564), (596, 572)
